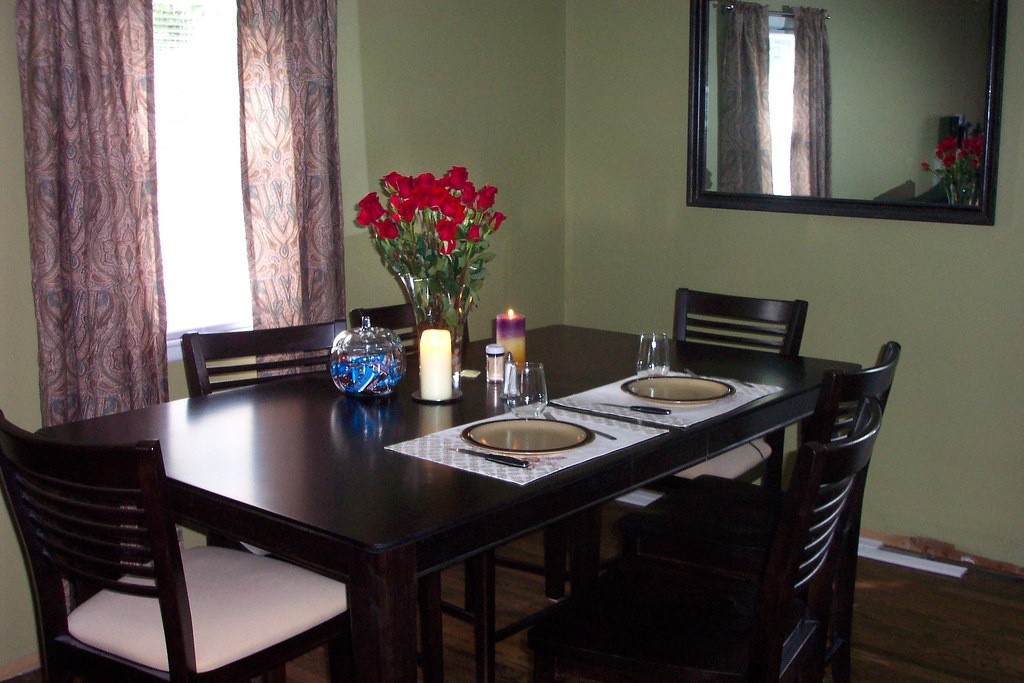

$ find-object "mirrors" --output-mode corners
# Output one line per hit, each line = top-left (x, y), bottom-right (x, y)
(686, 0), (1008, 225)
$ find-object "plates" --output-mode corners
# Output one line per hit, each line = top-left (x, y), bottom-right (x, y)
(412, 390), (463, 402)
(460, 419), (594, 455)
(621, 376), (736, 406)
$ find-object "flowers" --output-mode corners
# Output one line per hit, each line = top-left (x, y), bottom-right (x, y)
(356, 166), (507, 330)
(921, 133), (983, 205)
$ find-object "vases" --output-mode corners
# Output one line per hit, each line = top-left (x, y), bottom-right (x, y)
(944, 179), (981, 206)
(400, 273), (470, 377)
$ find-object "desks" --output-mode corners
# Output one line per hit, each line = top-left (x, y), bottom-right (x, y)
(32, 325), (862, 683)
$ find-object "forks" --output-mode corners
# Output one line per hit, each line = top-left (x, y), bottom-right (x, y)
(543, 412), (617, 440)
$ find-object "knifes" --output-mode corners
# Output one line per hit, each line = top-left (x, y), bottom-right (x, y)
(451, 449), (529, 468)
(595, 401), (671, 415)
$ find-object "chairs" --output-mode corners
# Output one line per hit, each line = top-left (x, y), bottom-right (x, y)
(349, 302), (567, 682)
(527, 398), (883, 683)
(181, 320), (443, 683)
(588, 286), (809, 583)
(612, 340), (901, 682)
(0, 411), (349, 683)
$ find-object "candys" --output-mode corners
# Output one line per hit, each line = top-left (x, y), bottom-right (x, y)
(329, 354), (404, 396)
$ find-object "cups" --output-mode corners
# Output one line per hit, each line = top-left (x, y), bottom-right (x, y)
(636, 333), (669, 378)
(506, 362), (548, 419)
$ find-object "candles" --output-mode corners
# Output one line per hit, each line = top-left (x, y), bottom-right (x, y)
(496, 308), (525, 364)
(419, 328), (451, 398)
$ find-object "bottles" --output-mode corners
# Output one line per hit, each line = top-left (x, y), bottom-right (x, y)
(330, 316), (406, 396)
(500, 352), (520, 398)
(486, 344), (506, 383)
